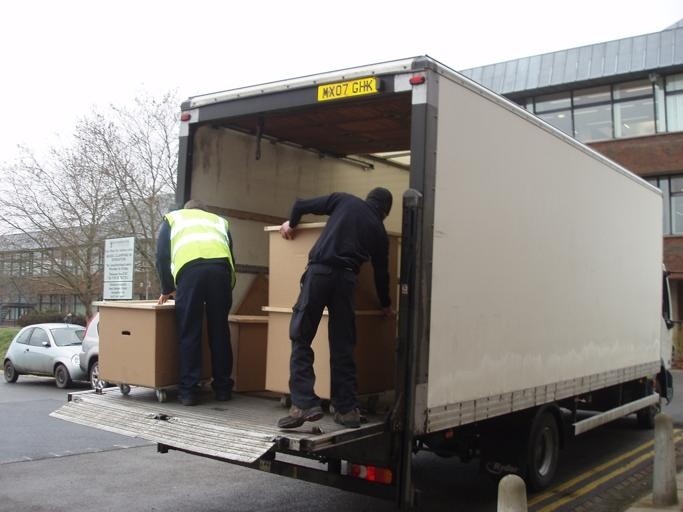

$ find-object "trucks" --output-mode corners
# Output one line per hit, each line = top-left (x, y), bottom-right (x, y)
(45, 52), (682, 512)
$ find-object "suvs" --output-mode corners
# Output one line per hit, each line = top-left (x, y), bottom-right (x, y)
(78, 312), (116, 389)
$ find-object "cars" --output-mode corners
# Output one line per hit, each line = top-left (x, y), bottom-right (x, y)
(3, 322), (89, 389)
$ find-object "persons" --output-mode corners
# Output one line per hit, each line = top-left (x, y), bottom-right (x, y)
(63, 312), (73, 324)
(277, 187), (396, 429)
(153, 198), (238, 407)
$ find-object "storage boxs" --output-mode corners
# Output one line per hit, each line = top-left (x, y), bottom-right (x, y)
(261, 306), (398, 399)
(91, 299), (211, 389)
(228, 315), (269, 392)
(263, 223), (402, 311)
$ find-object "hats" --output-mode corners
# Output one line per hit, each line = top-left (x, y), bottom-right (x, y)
(368, 187), (392, 214)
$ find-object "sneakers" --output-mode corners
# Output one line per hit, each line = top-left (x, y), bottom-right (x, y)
(278, 404), (323, 428)
(177, 390), (196, 405)
(215, 388), (232, 401)
(335, 407), (360, 428)
